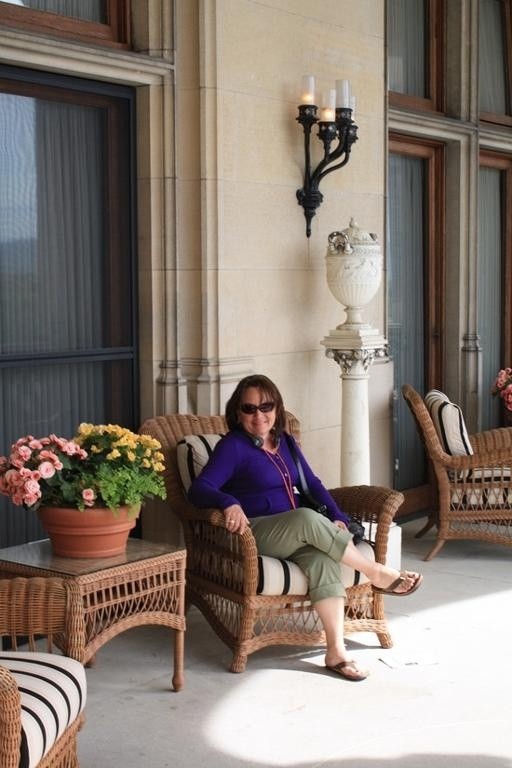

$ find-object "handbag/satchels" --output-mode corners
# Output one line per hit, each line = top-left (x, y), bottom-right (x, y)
(304, 496), (365, 544)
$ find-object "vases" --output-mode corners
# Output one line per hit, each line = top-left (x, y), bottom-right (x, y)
(37, 504), (141, 558)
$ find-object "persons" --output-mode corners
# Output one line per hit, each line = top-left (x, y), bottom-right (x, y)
(188, 375), (423, 681)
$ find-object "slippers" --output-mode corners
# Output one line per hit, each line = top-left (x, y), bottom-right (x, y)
(371, 571), (423, 596)
(327, 659), (369, 680)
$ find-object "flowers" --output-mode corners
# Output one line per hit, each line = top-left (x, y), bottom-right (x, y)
(0, 421), (168, 520)
(492, 367), (512, 411)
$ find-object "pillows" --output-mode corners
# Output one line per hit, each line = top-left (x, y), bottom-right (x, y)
(423, 389), (475, 478)
(176, 432), (230, 506)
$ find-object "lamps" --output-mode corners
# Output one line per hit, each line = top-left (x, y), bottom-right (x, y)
(296, 74), (359, 238)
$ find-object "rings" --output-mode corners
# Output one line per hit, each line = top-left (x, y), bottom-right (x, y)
(229, 520), (235, 524)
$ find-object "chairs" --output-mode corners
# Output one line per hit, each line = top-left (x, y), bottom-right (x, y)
(0, 576), (87, 768)
(402, 383), (512, 561)
(138, 410), (405, 674)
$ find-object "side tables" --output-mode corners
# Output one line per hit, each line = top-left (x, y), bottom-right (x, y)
(0, 535), (188, 693)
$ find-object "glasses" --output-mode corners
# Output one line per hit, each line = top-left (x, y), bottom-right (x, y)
(241, 401), (276, 413)
